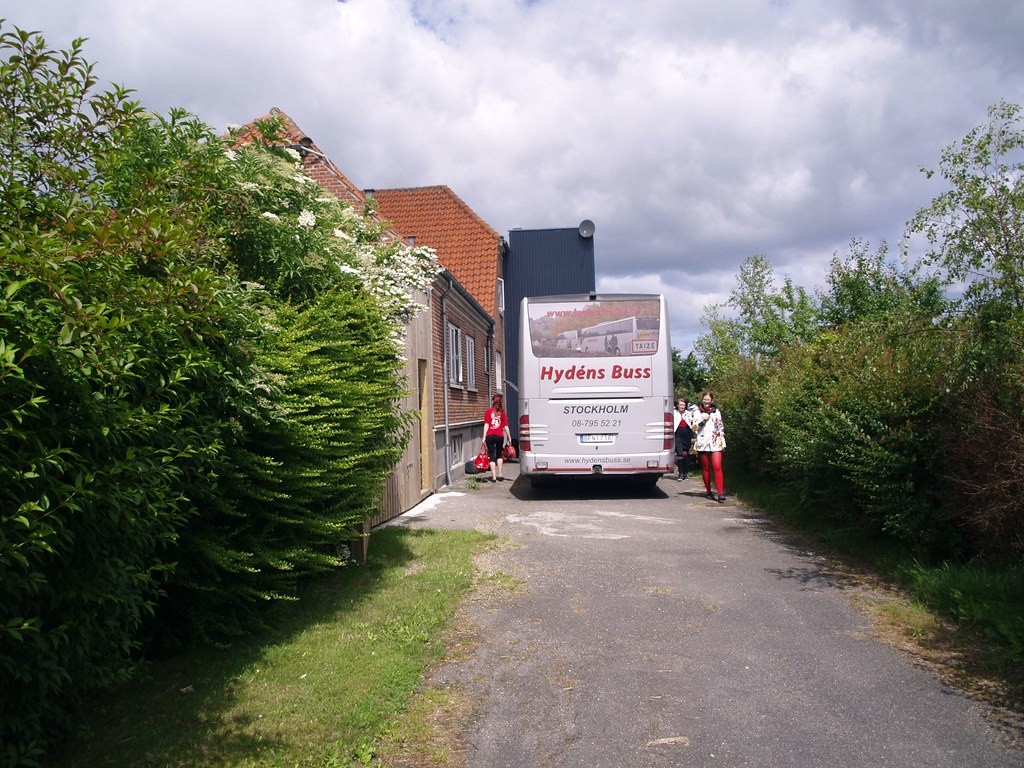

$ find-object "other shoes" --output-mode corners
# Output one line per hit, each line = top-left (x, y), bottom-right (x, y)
(682, 473), (689, 480)
(719, 495), (726, 502)
(707, 493), (716, 500)
(498, 477), (503, 481)
(677, 474), (683, 481)
(492, 478), (497, 482)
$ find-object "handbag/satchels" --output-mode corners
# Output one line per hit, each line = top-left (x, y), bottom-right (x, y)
(504, 441), (517, 458)
(474, 444), (489, 470)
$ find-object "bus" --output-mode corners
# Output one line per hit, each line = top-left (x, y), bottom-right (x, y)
(516, 293), (677, 479)
(553, 316), (660, 355)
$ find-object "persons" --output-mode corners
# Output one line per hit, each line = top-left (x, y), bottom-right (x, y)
(483, 395), (512, 482)
(692, 392), (727, 503)
(673, 398), (692, 481)
